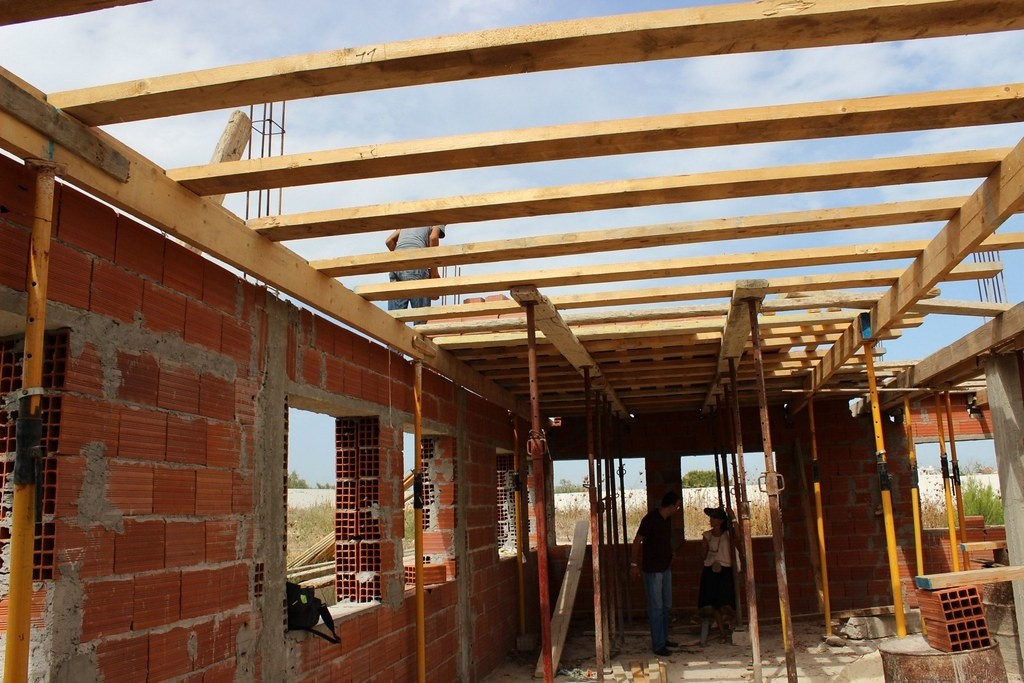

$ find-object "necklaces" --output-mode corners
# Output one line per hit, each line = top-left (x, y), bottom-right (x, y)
(707, 529), (724, 552)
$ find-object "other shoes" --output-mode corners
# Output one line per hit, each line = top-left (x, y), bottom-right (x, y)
(665, 639), (679, 648)
(655, 648), (672, 656)
(718, 632), (729, 644)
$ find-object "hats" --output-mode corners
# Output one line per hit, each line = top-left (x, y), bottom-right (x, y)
(704, 507), (727, 519)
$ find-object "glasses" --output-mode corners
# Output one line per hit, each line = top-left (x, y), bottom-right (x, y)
(672, 504), (681, 510)
(707, 543), (720, 553)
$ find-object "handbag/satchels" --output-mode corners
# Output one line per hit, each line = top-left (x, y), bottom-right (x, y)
(285, 580), (342, 645)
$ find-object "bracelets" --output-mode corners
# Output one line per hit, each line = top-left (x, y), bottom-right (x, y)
(631, 562), (638, 567)
(732, 518), (737, 522)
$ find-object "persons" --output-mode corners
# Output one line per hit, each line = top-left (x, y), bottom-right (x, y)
(700, 507), (746, 644)
(631, 494), (683, 656)
(385, 223), (445, 356)
